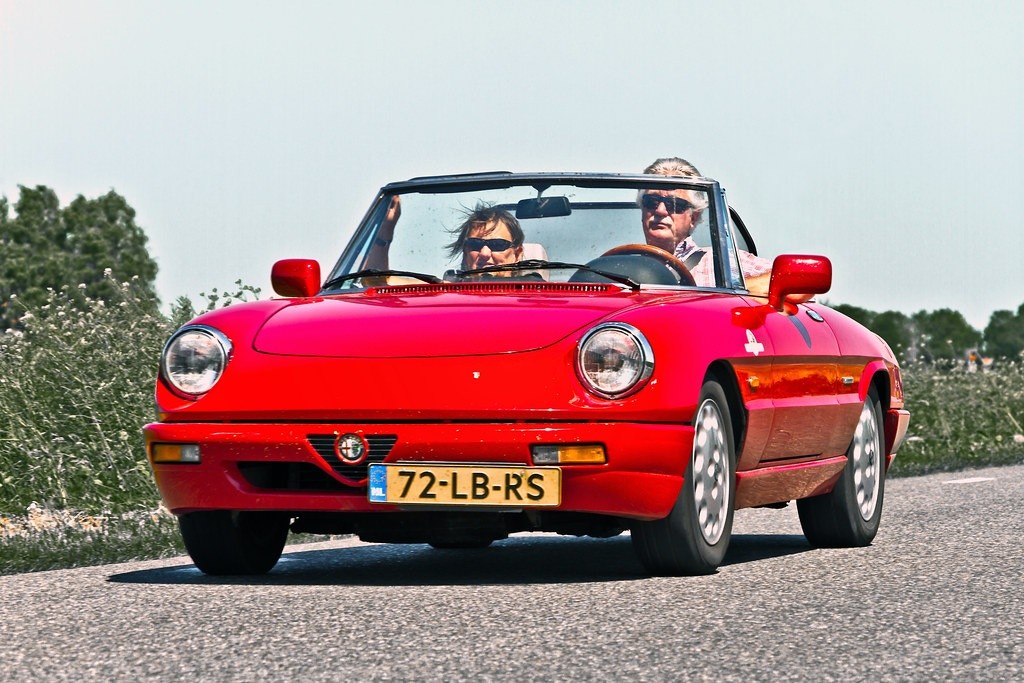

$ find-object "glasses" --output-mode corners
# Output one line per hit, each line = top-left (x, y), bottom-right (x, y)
(642, 193), (694, 214)
(465, 237), (517, 252)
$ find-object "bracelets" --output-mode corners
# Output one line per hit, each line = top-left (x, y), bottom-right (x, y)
(375, 238), (394, 248)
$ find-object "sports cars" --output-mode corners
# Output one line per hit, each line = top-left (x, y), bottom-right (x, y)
(140, 170), (911, 581)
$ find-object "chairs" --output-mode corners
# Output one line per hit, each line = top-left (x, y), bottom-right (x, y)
(448, 243), (551, 283)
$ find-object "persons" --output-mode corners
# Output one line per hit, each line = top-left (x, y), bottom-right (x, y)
(359, 194), (551, 291)
(636, 158), (815, 305)
